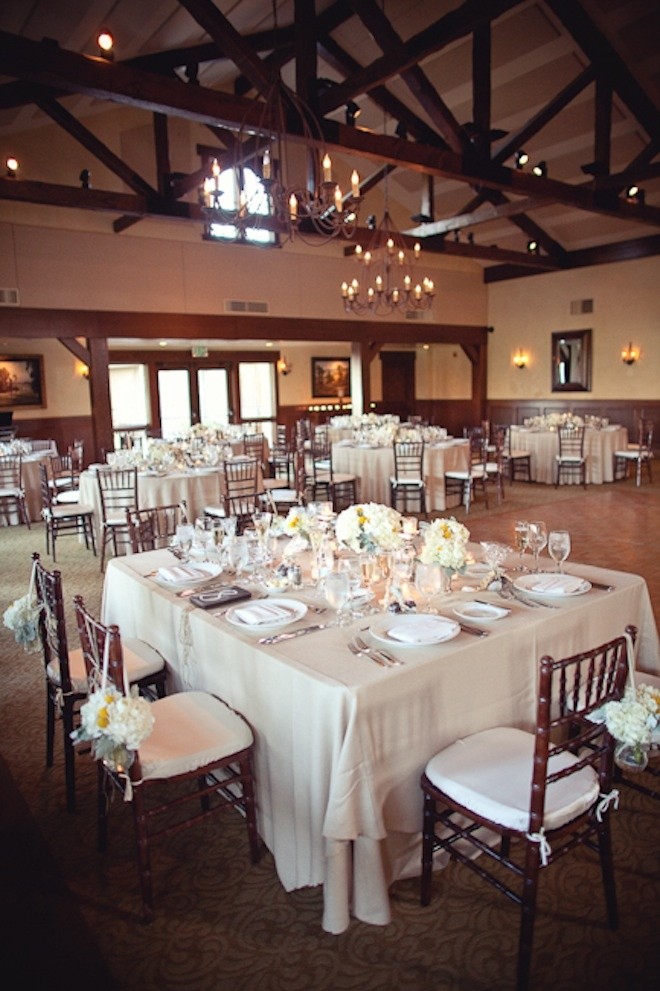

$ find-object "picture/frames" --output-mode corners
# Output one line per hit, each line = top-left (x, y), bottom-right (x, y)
(0, 353), (47, 411)
(311, 357), (351, 398)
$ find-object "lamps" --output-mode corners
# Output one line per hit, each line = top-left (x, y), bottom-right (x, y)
(198, 78), (548, 317)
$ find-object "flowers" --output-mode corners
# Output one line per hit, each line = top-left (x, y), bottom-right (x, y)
(285, 505), (332, 544)
(3, 559), (54, 656)
(421, 519), (471, 570)
(70, 633), (154, 772)
(585, 635), (660, 773)
(337, 500), (403, 555)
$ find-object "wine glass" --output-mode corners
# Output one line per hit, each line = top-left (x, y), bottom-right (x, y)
(176, 502), (440, 626)
(529, 521), (548, 574)
(547, 530), (571, 574)
(514, 521), (529, 572)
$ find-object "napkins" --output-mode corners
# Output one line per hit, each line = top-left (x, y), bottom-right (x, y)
(159, 565), (205, 581)
(389, 620), (457, 643)
(532, 574), (585, 593)
(235, 604), (292, 624)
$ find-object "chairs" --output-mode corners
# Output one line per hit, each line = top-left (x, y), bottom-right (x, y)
(0, 432), (654, 991)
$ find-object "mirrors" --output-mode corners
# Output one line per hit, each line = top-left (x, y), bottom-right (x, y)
(552, 329), (591, 392)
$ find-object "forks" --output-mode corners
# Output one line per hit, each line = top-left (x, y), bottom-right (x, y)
(346, 636), (399, 668)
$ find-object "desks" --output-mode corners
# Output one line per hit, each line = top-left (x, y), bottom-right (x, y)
(0, 450), (59, 528)
(78, 460), (264, 556)
(332, 439), (470, 512)
(505, 425), (628, 485)
(102, 548), (660, 935)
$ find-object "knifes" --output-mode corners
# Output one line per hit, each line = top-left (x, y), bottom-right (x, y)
(259, 625), (324, 644)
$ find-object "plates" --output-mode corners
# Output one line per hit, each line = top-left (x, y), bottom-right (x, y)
(370, 614), (460, 647)
(226, 597), (309, 631)
(512, 573), (591, 597)
(465, 564), (492, 579)
(157, 563), (223, 586)
(453, 602), (507, 622)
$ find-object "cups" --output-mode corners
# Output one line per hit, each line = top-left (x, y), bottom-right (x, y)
(0, 437), (32, 454)
(331, 414), (446, 446)
(523, 412), (608, 432)
(107, 437), (233, 480)
